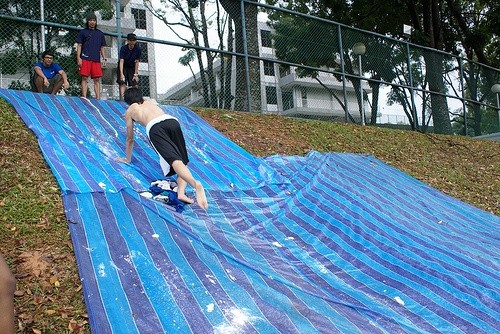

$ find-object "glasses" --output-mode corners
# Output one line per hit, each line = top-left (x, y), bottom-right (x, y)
(45, 56), (53, 59)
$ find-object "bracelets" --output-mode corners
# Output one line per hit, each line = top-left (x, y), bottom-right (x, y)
(103, 58), (108, 62)
(134, 74), (138, 77)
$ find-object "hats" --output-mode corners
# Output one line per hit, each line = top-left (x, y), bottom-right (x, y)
(126, 33), (137, 39)
(86, 14), (96, 20)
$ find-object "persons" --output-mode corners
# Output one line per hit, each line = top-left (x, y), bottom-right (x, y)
(32, 50), (69, 95)
(116, 33), (141, 101)
(74, 12), (108, 99)
(113, 87), (208, 209)
